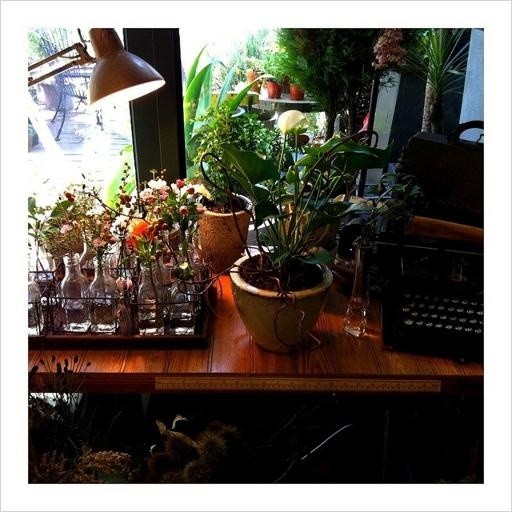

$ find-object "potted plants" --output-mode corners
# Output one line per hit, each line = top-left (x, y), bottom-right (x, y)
(342, 172), (423, 337)
(188, 100), (282, 274)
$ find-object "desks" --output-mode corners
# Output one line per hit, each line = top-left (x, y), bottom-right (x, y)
(28, 271), (484, 444)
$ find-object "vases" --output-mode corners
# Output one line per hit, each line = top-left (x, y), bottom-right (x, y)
(28, 223), (197, 334)
(290, 83), (304, 100)
(265, 79), (281, 98)
(229, 253), (334, 354)
(246, 70), (265, 94)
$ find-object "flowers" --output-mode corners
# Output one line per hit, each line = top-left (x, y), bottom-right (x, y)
(177, 109), (395, 277)
(371, 29), (470, 134)
(29, 161), (207, 320)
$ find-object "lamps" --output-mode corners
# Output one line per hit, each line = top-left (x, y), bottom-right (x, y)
(28, 28), (167, 106)
